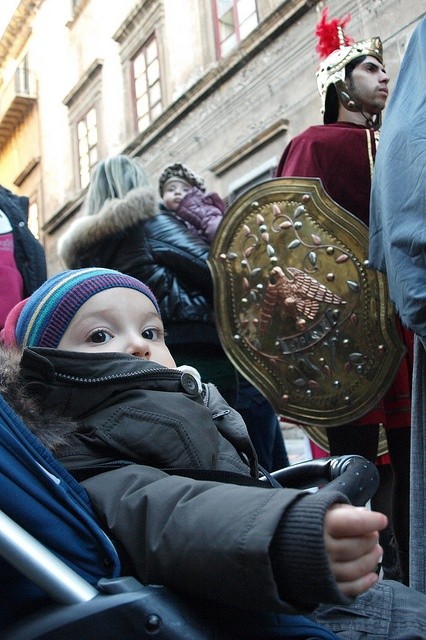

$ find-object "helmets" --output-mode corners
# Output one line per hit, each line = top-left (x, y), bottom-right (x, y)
(315, 26), (385, 125)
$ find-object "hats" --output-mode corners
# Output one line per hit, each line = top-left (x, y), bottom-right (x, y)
(158, 162), (206, 193)
(0, 265), (162, 352)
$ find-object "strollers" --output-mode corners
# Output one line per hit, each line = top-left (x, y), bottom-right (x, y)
(0, 391), (426, 640)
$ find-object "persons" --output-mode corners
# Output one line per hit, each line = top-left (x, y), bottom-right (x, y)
(262, 36), (414, 586)
(0, 266), (426, 640)
(157, 161), (225, 243)
(57, 154), (290, 474)
(0, 187), (47, 335)
